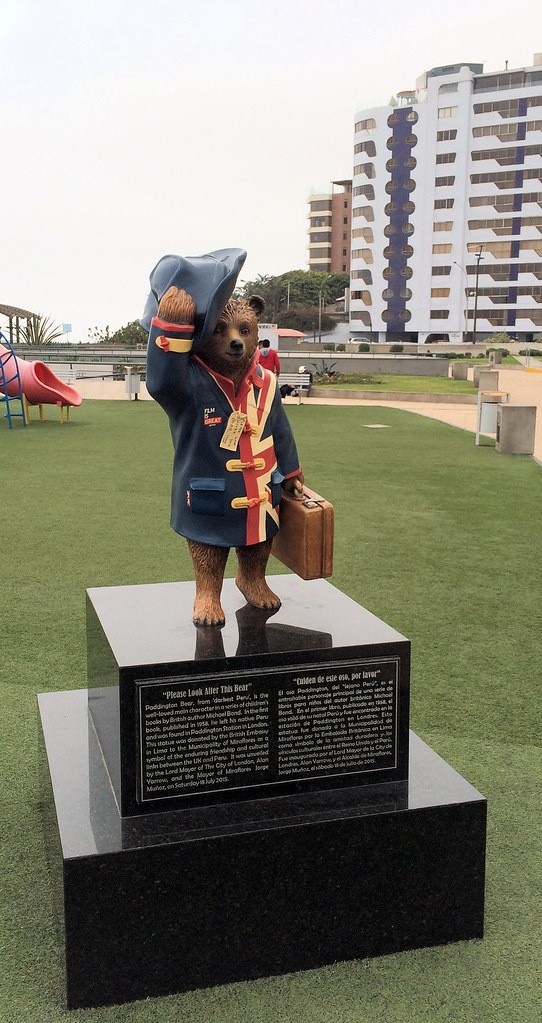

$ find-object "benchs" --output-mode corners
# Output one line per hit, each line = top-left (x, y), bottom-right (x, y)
(51, 368), (77, 387)
(277, 372), (311, 406)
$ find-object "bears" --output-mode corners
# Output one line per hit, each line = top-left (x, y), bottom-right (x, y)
(145, 285), (306, 629)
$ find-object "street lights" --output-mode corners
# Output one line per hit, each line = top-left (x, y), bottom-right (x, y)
(469, 244), (484, 344)
(454, 262), (469, 343)
(318, 274), (332, 343)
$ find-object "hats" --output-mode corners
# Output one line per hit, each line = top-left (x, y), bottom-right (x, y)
(298, 366), (306, 373)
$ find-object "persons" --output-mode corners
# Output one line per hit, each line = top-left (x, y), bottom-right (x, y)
(255, 340), (264, 365)
(426, 349), (434, 358)
(291, 365), (314, 397)
(258, 339), (281, 377)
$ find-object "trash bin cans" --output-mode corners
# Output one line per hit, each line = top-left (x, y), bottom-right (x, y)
(474, 389), (510, 445)
